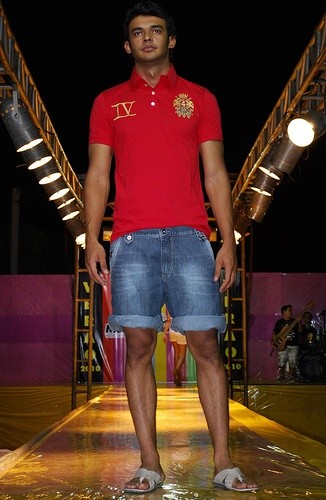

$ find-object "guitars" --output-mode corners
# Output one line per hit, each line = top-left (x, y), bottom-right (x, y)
(272, 300), (313, 349)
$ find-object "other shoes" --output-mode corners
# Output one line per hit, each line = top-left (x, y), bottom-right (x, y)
(173, 369), (183, 387)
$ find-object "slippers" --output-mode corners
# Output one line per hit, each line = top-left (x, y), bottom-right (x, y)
(124, 468), (164, 493)
(212, 466), (259, 492)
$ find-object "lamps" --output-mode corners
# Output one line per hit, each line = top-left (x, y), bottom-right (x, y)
(53, 191), (76, 210)
(59, 201), (80, 221)
(250, 169), (278, 197)
(33, 159), (62, 185)
(287, 109), (325, 149)
(21, 131), (56, 171)
(246, 191), (274, 224)
(258, 111), (306, 181)
(42, 176), (70, 201)
(0, 84), (45, 154)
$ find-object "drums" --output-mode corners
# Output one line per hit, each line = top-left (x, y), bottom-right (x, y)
(295, 351), (326, 382)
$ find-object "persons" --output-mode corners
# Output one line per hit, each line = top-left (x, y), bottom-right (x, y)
(300, 311), (317, 353)
(271, 304), (299, 386)
(162, 310), (188, 387)
(84, 4), (260, 494)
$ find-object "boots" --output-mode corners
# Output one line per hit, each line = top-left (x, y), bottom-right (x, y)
(291, 367), (303, 382)
(272, 367), (286, 384)
